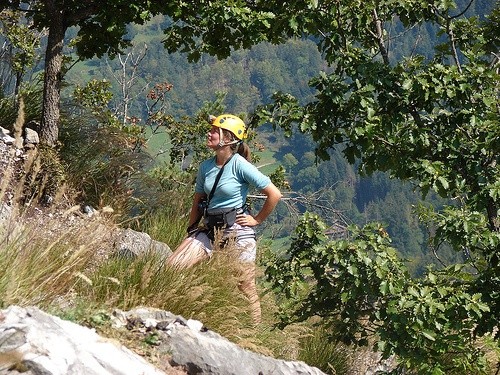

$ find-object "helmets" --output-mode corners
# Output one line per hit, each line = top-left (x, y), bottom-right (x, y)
(209, 113), (248, 147)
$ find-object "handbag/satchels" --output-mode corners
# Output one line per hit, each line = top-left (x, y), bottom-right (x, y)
(207, 207), (237, 230)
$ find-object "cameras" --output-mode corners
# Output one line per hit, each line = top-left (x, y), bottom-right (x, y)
(197, 197), (207, 216)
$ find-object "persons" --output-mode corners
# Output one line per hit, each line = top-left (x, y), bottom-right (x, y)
(164, 113), (282, 329)
